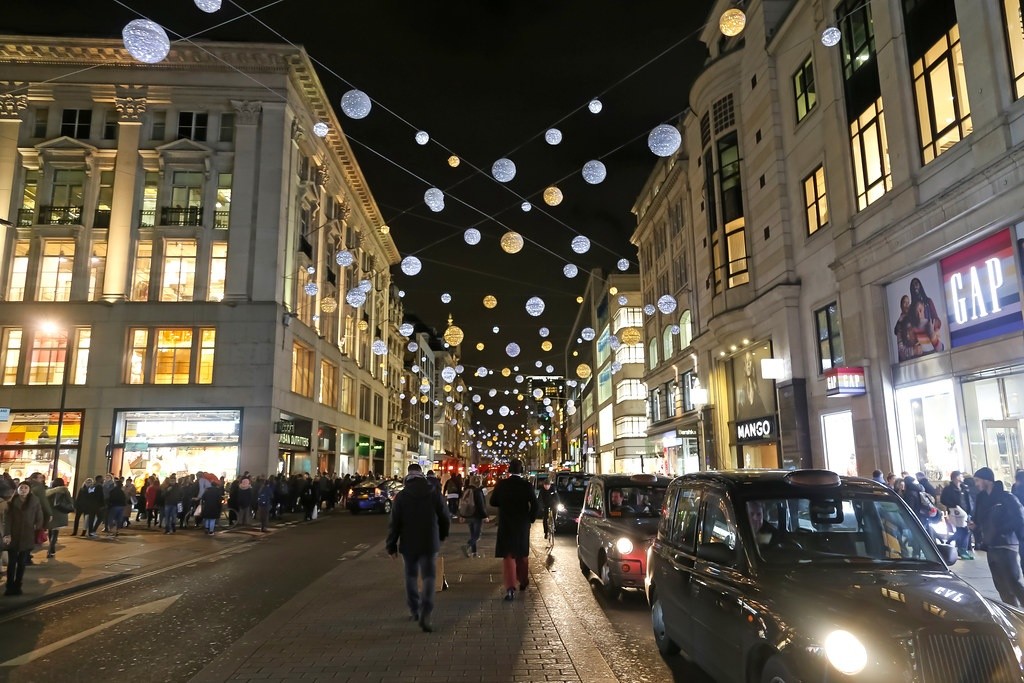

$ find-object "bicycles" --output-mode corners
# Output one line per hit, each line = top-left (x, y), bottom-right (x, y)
(184, 497), (240, 530)
(547, 507), (556, 546)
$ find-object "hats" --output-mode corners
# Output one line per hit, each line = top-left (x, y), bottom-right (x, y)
(904, 476), (915, 485)
(974, 467), (994, 481)
(543, 479), (551, 485)
(242, 479), (249, 484)
(508, 461), (524, 474)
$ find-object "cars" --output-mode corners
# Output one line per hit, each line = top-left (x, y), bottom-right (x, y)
(642, 466), (1024, 683)
(346, 479), (404, 514)
(550, 471), (599, 533)
(481, 473), (548, 516)
(575, 474), (678, 598)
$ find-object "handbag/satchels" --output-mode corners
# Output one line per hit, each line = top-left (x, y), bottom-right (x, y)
(311, 505), (318, 520)
(34, 528), (47, 544)
(194, 505), (202, 517)
(948, 505), (967, 527)
(130, 495), (138, 504)
(417, 556), (444, 592)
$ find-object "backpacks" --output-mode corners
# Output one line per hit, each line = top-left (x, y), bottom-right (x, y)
(256, 494), (268, 506)
(458, 487), (477, 517)
(54, 491), (74, 514)
(918, 491), (937, 518)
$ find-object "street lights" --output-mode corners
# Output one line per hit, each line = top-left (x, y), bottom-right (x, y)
(98, 435), (112, 475)
(39, 318), (73, 486)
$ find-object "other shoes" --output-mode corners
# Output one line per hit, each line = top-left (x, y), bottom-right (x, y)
(472, 553), (478, 558)
(461, 544), (470, 557)
(418, 611), (435, 632)
(69, 519), (266, 536)
(47, 550), (56, 557)
(519, 579), (529, 591)
(941, 541), (982, 560)
(410, 608), (420, 620)
(505, 589), (516, 600)
(4, 582), (22, 595)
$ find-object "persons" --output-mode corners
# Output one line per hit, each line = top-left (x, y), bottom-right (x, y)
(611, 489), (635, 511)
(489, 458), (538, 600)
(734, 348), (772, 421)
(891, 276), (948, 361)
(560, 477), (581, 492)
(443, 469), (490, 558)
(385, 463), (451, 632)
(869, 466), (1024, 608)
(724, 500), (792, 547)
(539, 479), (559, 539)
(0, 468), (402, 597)
